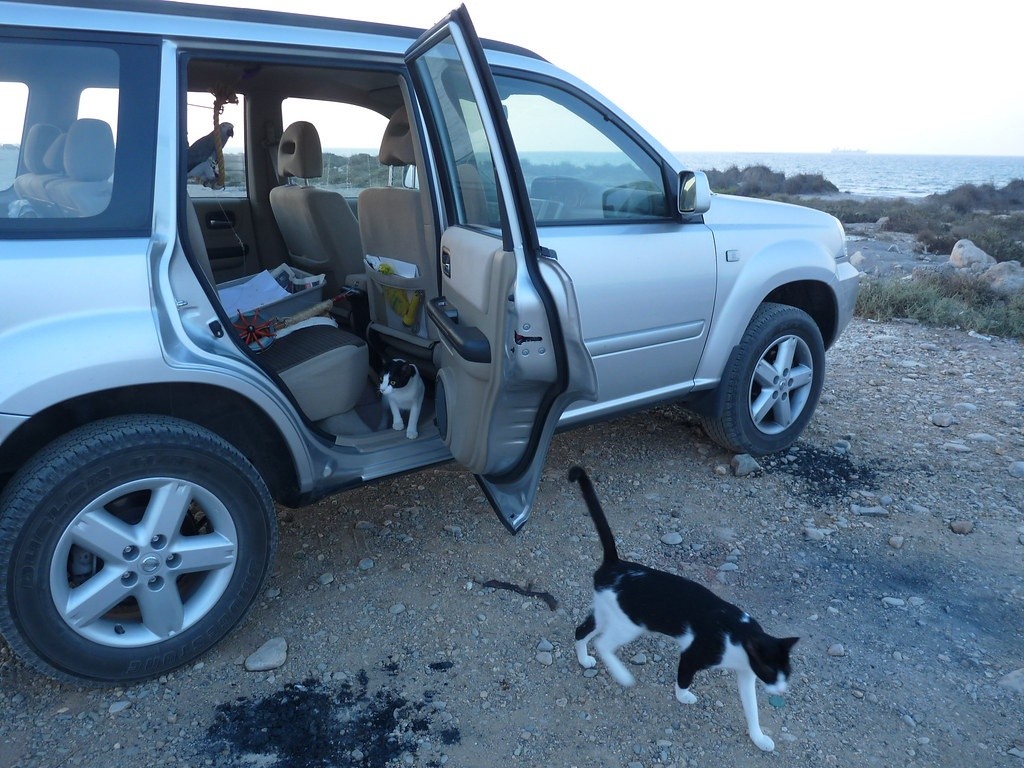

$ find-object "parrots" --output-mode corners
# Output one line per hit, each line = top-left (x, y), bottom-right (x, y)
(187, 122), (235, 191)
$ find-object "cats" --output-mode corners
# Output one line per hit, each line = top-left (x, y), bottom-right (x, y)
(379, 356), (426, 440)
(567, 464), (800, 753)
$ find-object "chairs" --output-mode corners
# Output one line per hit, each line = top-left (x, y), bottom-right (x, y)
(12, 105), (443, 424)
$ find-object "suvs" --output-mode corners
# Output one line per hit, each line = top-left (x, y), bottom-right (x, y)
(0, 0), (861, 688)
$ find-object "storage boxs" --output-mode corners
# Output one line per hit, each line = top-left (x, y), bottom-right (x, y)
(216, 267), (327, 345)
